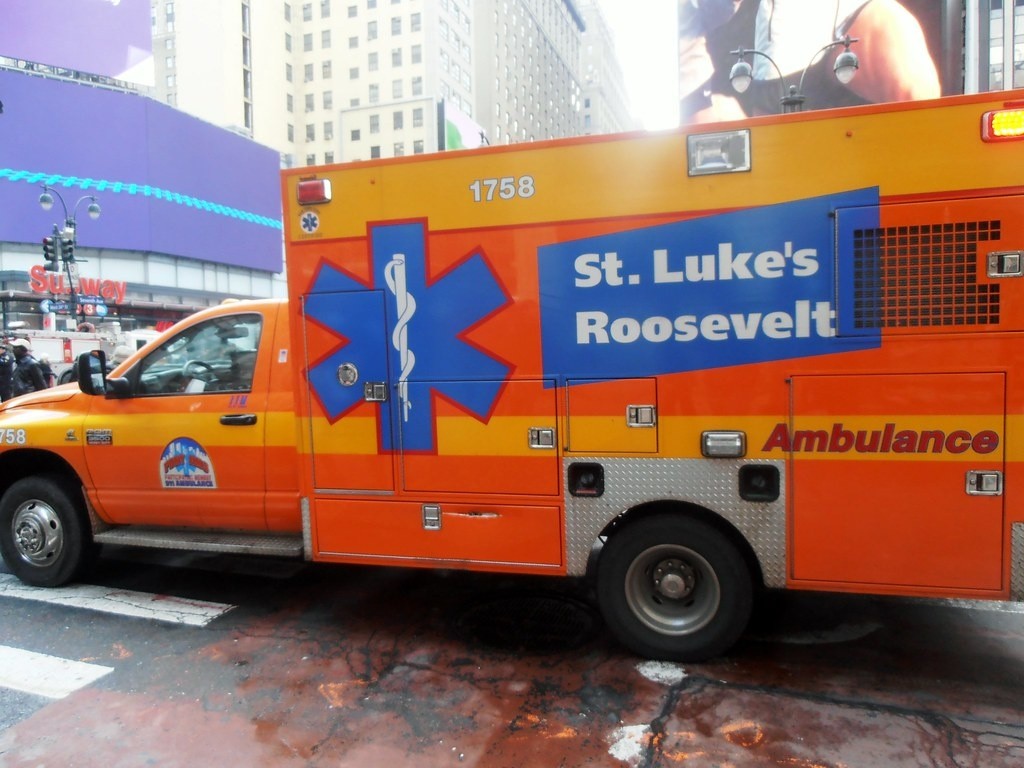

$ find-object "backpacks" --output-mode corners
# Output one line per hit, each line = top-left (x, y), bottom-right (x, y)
(29, 357), (52, 389)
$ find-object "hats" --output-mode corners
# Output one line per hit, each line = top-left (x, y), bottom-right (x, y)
(112, 345), (134, 363)
(9, 339), (30, 351)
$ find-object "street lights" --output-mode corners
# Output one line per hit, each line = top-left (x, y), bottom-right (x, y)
(728, 37), (861, 115)
(37, 182), (101, 332)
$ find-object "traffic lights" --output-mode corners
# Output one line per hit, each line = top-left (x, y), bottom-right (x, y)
(59, 238), (73, 263)
(43, 236), (59, 271)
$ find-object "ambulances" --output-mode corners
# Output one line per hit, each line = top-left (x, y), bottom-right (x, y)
(1, 91), (1022, 666)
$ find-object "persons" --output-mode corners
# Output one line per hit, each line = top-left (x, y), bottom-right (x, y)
(678, 0), (942, 126)
(0, 336), (58, 403)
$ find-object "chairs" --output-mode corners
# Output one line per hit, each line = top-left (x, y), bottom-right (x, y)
(229, 348), (258, 388)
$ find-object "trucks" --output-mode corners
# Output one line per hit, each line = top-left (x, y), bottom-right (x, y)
(6, 330), (187, 387)
